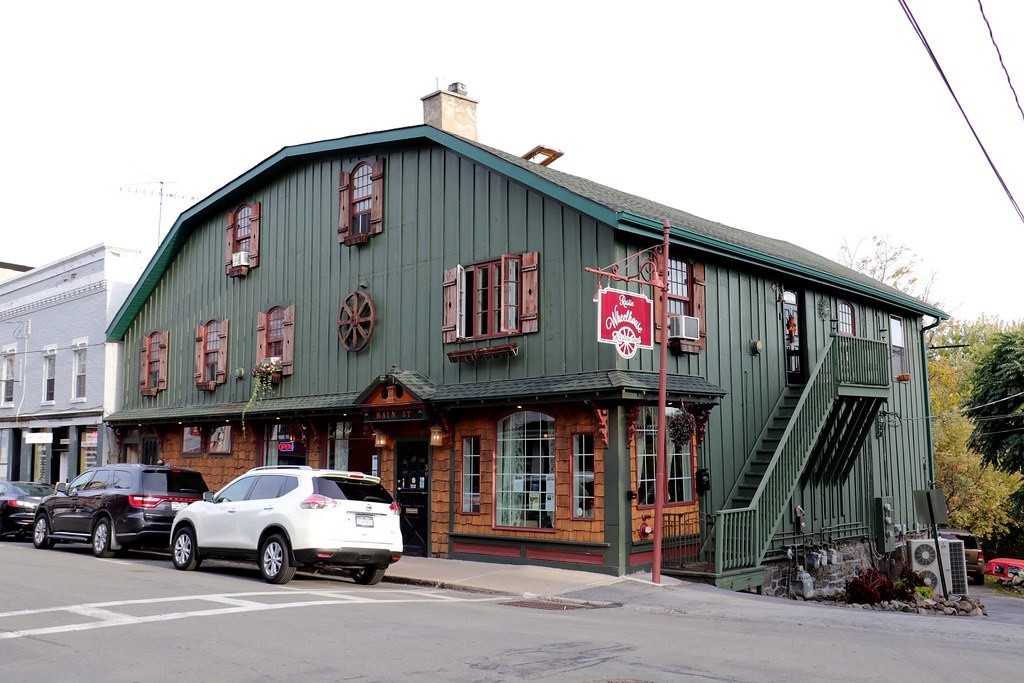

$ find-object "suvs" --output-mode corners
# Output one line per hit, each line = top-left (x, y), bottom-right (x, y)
(169, 465), (404, 584)
(498, 472), (595, 521)
(938, 527), (985, 585)
(33, 463), (210, 559)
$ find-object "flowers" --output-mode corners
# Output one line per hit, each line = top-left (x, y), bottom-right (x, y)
(241, 362), (285, 436)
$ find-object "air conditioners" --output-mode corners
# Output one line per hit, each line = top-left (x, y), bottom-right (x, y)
(231, 251), (251, 267)
(906, 539), (969, 597)
(669, 315), (700, 341)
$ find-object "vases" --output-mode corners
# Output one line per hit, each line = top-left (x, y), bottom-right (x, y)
(446, 343), (520, 363)
(343, 233), (369, 248)
(228, 265), (249, 277)
(669, 339), (700, 357)
(258, 370), (281, 384)
(196, 381), (216, 391)
(140, 388), (157, 396)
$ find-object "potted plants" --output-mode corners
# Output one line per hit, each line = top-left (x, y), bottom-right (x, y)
(668, 397), (700, 453)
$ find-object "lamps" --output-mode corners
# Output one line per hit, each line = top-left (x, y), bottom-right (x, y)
(429, 422), (448, 446)
(374, 427), (392, 447)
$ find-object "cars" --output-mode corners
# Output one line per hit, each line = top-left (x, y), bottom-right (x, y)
(0, 480), (55, 539)
